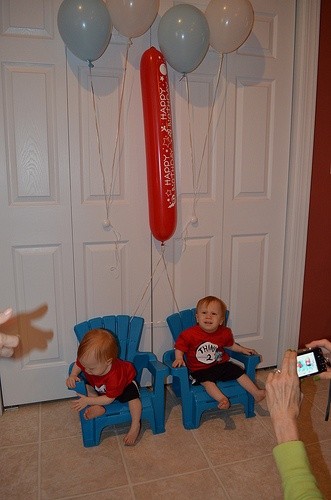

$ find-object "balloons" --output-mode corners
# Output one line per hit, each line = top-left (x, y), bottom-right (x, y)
(106, 0), (160, 38)
(158, 4), (209, 74)
(56, 0), (111, 61)
(140, 47), (177, 241)
(205, 0), (254, 56)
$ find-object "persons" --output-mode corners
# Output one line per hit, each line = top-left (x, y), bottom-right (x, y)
(264, 338), (331, 500)
(66, 328), (142, 444)
(295, 357), (311, 366)
(0, 308), (18, 415)
(172, 296), (266, 410)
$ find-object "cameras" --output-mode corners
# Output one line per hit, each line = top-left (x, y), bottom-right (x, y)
(295, 346), (329, 379)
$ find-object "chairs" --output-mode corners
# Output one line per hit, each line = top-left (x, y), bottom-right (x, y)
(163, 308), (260, 429)
(68, 315), (170, 448)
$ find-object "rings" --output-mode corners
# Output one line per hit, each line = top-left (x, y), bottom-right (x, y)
(273, 369), (280, 374)
